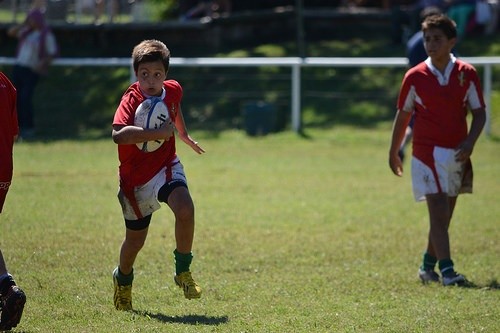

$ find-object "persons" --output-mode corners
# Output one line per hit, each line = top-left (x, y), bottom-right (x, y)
(398, 7), (460, 165)
(0, 71), (27, 333)
(8, 8), (58, 139)
(388, 15), (487, 288)
(112, 38), (206, 314)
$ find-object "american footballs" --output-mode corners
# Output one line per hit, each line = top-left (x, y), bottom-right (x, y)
(135, 98), (170, 152)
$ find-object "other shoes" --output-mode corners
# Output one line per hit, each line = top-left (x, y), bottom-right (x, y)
(398, 150), (404, 163)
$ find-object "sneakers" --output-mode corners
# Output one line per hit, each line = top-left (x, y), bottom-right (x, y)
(0, 284), (27, 330)
(112, 267), (133, 312)
(174, 271), (202, 300)
(442, 272), (465, 286)
(418, 268), (440, 285)
